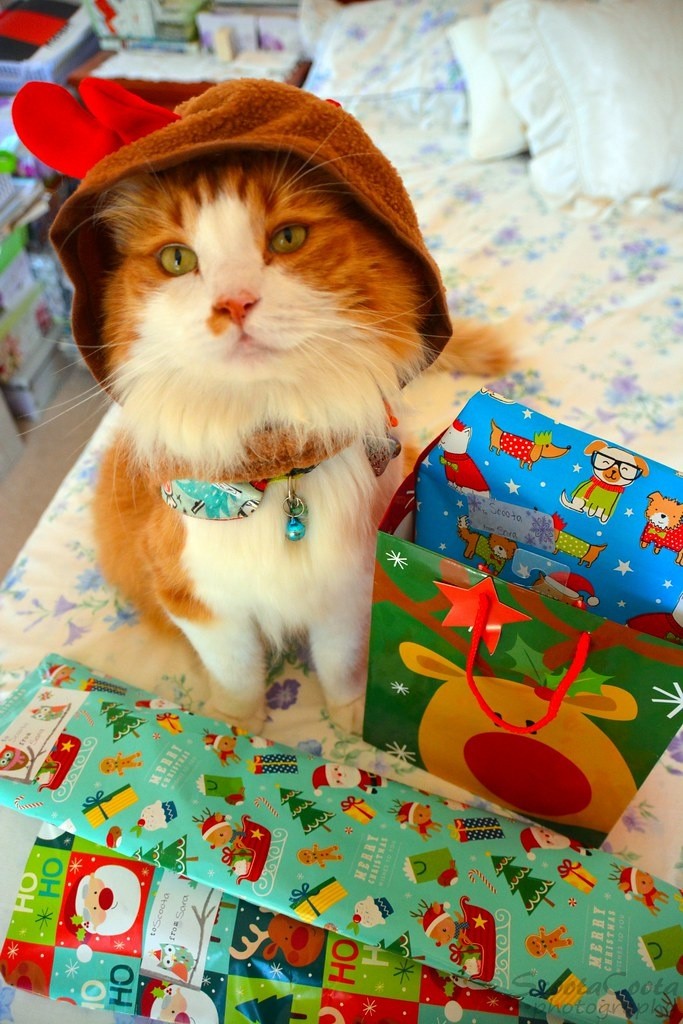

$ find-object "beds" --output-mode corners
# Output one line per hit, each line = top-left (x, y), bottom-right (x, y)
(1, 1), (682, 1024)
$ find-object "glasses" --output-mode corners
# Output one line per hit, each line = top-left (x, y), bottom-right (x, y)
(591, 450), (644, 480)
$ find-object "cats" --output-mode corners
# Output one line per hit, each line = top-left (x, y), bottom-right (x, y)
(90, 153), (517, 736)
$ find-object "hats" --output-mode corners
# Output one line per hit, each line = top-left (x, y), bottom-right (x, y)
(42, 665), (67, 684)
(620, 867), (638, 901)
(311, 763), (335, 796)
(421, 901), (451, 938)
(11, 75), (455, 408)
(202, 733), (223, 750)
(519, 826), (545, 861)
(201, 813), (232, 839)
(134, 699), (152, 711)
(399, 802), (419, 830)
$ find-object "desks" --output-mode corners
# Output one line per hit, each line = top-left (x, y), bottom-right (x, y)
(61, 43), (317, 103)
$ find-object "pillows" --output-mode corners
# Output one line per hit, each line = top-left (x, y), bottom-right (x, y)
(483, 2), (683, 206)
(444, 5), (527, 163)
